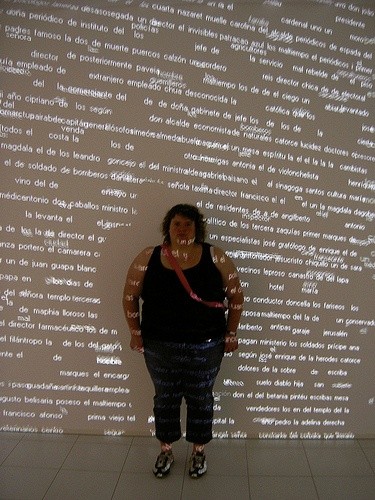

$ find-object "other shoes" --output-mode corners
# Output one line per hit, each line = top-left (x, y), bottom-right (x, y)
(189, 447), (207, 479)
(152, 448), (174, 479)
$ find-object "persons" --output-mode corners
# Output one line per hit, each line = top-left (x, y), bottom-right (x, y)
(123, 202), (244, 479)
